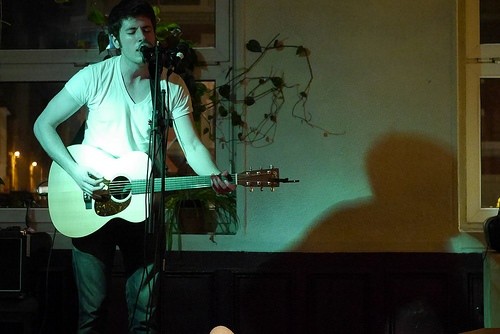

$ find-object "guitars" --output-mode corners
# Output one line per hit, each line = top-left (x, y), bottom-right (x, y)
(47, 143), (299, 239)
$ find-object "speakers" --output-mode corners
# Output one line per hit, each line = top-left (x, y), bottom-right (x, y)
(0, 232), (53, 298)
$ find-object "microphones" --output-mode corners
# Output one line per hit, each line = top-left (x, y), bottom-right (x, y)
(166, 42), (188, 74)
(139, 41), (153, 61)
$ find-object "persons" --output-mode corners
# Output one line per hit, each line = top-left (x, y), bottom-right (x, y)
(32, 0), (238, 334)
